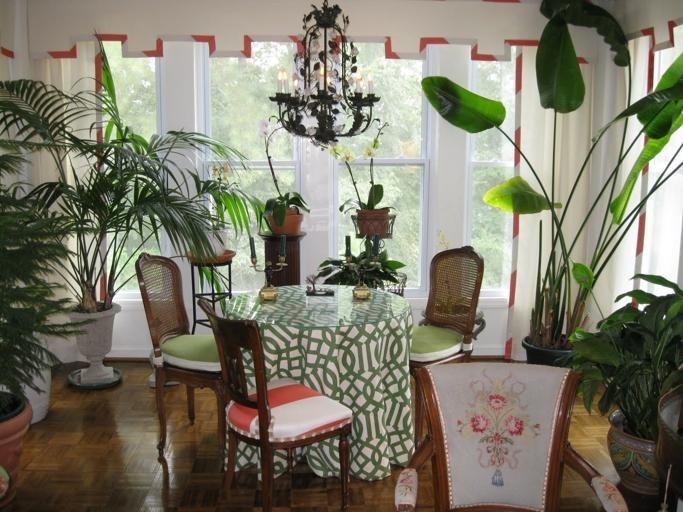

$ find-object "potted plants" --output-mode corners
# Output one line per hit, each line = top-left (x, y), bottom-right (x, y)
(0, 142), (99, 507)
(421, 0), (683, 367)
(554, 259), (683, 494)
(0, 34), (266, 382)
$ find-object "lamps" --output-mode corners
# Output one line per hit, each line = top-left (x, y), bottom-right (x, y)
(268, 0), (381, 144)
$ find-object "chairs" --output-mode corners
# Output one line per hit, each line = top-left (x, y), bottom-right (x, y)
(136, 251), (228, 458)
(197, 298), (353, 511)
(394, 355), (629, 511)
(409, 245), (484, 444)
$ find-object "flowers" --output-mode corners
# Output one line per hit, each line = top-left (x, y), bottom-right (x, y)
(258, 118), (311, 227)
(204, 162), (232, 228)
(328, 120), (398, 214)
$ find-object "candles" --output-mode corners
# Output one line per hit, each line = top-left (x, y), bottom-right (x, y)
(249, 236), (256, 259)
(346, 235), (351, 258)
(373, 232), (380, 255)
(280, 234), (286, 257)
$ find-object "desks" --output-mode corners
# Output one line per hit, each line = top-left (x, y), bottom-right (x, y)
(421, 308), (486, 340)
(224, 283), (415, 481)
(184, 249), (236, 336)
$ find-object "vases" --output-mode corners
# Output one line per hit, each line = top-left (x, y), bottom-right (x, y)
(268, 207), (304, 235)
(189, 228), (228, 256)
(356, 208), (390, 235)
